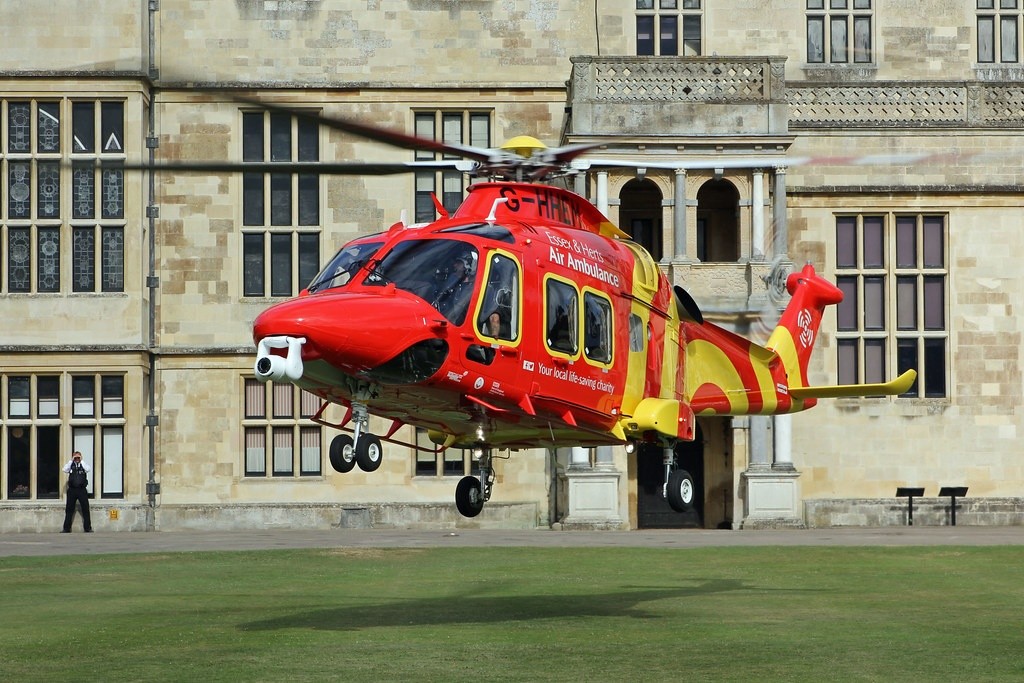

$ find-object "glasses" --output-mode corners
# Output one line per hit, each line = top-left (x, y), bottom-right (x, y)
(74, 457), (80, 460)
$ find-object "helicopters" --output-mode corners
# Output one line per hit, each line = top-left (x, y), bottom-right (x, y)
(41, 62), (980, 520)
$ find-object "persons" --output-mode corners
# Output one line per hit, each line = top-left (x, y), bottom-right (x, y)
(483, 313), (501, 338)
(58, 451), (94, 533)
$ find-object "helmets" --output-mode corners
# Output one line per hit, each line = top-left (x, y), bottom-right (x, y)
(455, 250), (478, 275)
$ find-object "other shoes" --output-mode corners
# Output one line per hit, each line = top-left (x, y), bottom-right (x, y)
(84, 529), (94, 533)
(60, 530), (72, 533)
